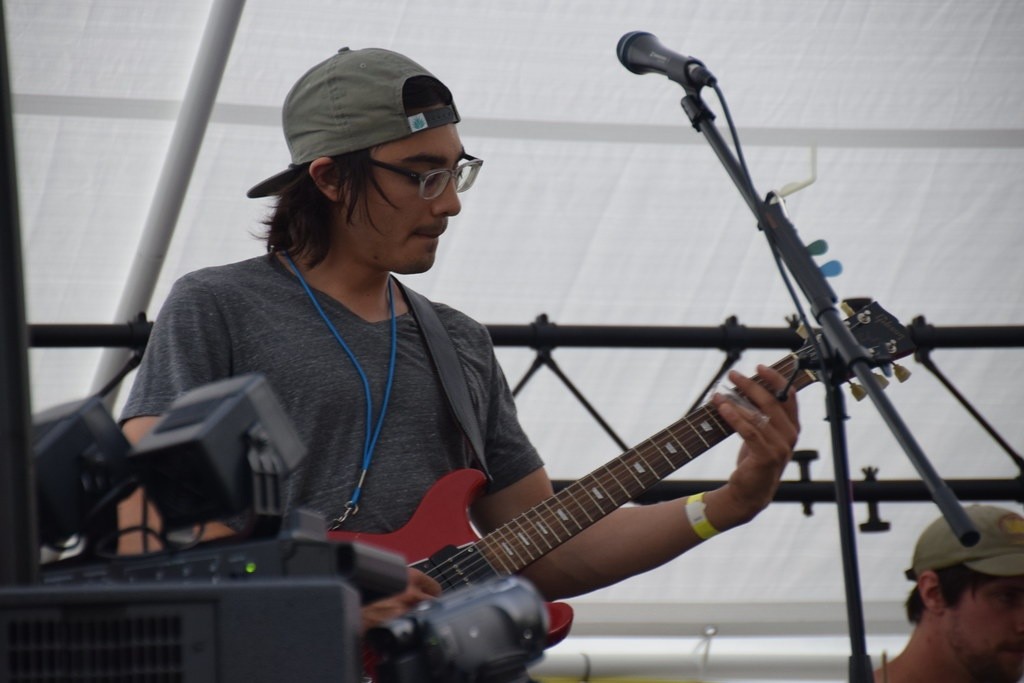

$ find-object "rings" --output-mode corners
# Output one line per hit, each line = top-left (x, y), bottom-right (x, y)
(755, 415), (772, 431)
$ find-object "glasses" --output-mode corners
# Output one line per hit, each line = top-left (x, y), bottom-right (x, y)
(371, 152), (483, 199)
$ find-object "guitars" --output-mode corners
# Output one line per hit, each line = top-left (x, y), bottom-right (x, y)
(328, 296), (921, 683)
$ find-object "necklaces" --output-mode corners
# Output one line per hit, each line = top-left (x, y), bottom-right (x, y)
(284, 249), (396, 530)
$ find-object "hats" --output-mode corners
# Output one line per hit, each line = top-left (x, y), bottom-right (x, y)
(905, 505), (1024, 579)
(246, 46), (461, 199)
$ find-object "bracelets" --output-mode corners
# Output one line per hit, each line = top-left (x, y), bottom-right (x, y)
(685, 491), (722, 540)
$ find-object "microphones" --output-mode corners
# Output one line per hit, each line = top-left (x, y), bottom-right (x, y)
(616, 31), (717, 86)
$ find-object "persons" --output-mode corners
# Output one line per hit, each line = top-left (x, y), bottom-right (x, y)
(873, 503), (1024, 683)
(116, 45), (801, 629)
